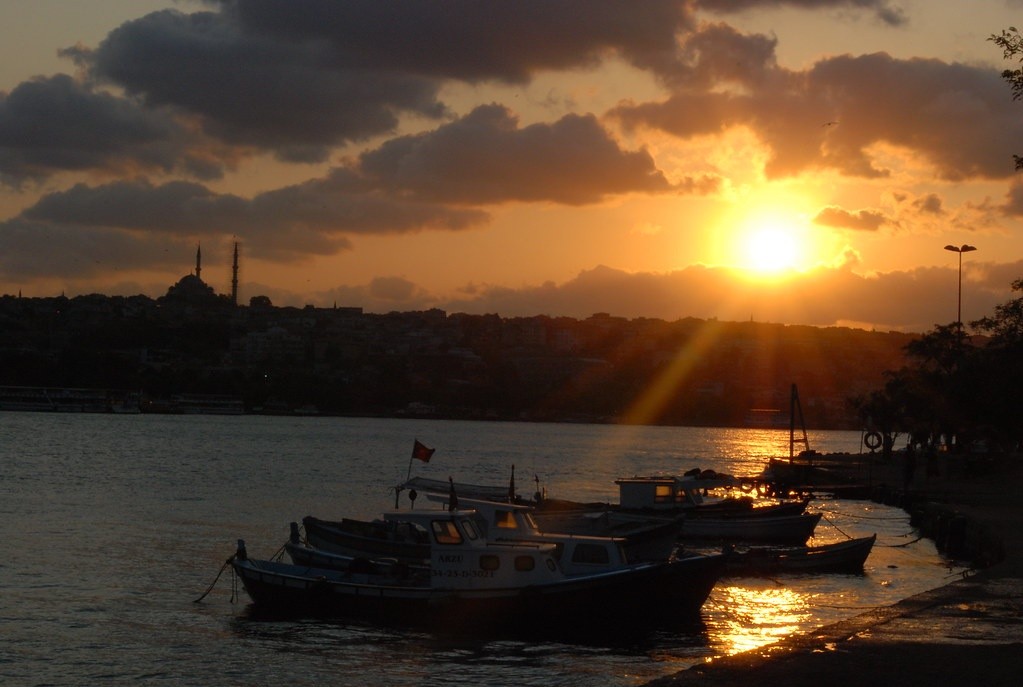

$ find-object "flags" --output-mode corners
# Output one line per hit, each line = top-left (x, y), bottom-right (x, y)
(412, 440), (435, 463)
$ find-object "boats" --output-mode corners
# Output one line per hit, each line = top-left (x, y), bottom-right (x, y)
(544, 494), (814, 516)
(674, 532), (880, 577)
(226, 509), (742, 622)
(393, 475), (823, 548)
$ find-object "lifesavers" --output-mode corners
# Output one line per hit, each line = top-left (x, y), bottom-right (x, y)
(864, 431), (882, 449)
(722, 475), (735, 489)
(739, 479), (775, 496)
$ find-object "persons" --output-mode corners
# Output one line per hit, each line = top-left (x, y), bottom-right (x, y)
(903, 444), (917, 490)
(926, 447), (939, 484)
